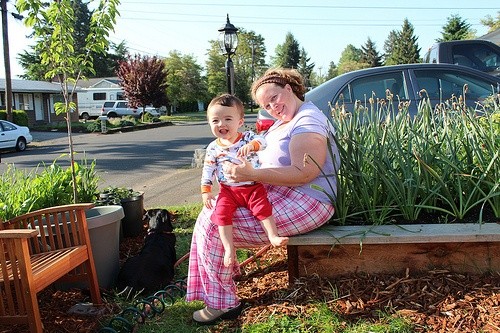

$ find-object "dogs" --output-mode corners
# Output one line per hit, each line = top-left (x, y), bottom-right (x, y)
(114, 208), (179, 303)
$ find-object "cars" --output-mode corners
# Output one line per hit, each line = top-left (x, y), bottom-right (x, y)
(255, 62), (500, 137)
(0, 119), (34, 151)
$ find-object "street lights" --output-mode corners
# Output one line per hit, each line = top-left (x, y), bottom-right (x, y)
(217, 13), (239, 96)
(250, 42), (258, 112)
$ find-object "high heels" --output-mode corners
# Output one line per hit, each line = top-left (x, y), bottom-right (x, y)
(192, 296), (242, 324)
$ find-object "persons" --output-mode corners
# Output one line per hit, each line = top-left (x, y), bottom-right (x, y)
(200, 94), (289, 267)
(184, 68), (342, 324)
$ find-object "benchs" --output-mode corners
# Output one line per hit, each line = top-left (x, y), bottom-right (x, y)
(281, 224), (500, 288)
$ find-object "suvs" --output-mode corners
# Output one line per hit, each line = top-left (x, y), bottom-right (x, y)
(100, 100), (162, 121)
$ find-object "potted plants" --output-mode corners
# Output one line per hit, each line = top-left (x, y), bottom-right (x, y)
(12, 0), (122, 291)
(103, 187), (146, 240)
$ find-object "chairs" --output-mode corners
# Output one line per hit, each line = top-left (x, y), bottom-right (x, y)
(0, 202), (101, 333)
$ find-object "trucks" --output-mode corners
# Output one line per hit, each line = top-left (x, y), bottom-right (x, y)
(422, 39), (500, 81)
(77, 87), (129, 121)
